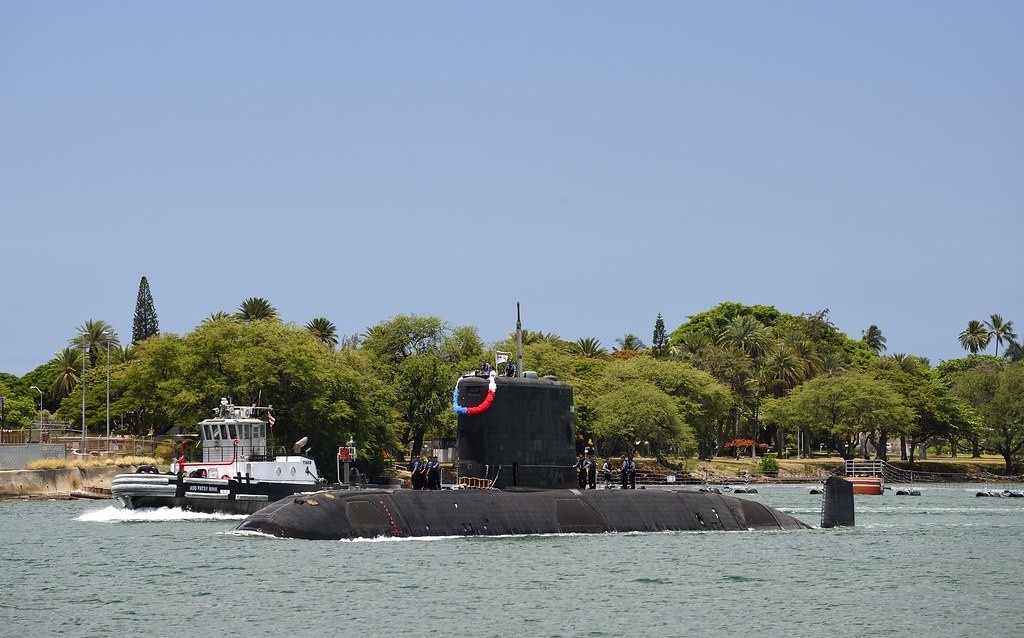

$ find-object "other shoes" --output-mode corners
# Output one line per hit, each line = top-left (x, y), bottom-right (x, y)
(605, 486), (610, 489)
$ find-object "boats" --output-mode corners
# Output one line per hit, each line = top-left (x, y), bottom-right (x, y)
(111, 390), (356, 516)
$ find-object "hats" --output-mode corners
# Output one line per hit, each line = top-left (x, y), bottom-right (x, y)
(621, 455), (625, 458)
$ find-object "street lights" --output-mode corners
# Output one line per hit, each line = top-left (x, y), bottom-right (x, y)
(81, 332), (89, 452)
(103, 331), (118, 458)
(29, 386), (43, 443)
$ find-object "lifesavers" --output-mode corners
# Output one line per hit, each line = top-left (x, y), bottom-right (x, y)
(135, 465), (160, 476)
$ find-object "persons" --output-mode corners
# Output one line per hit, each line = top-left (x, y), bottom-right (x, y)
(573, 454), (596, 489)
(603, 458), (612, 490)
(411, 455), (439, 490)
(489, 366), (496, 376)
(620, 455), (636, 489)
(481, 359), (491, 373)
(505, 361), (516, 377)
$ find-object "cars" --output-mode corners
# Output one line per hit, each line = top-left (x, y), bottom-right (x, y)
(886, 443), (892, 450)
